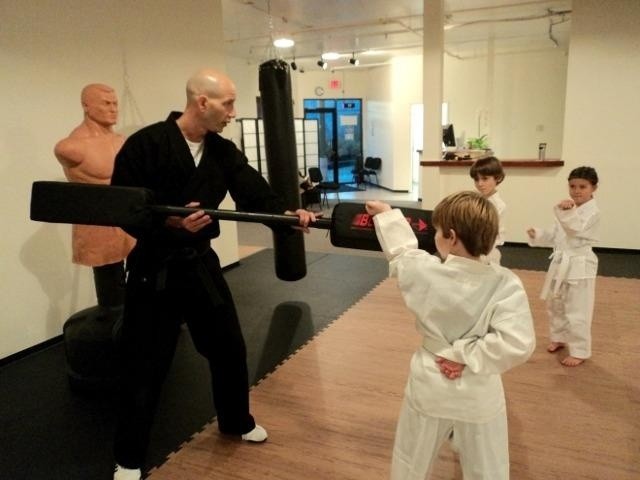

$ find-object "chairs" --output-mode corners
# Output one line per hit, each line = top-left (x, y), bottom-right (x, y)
(300, 157), (382, 210)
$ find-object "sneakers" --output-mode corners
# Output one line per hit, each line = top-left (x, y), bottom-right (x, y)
(113, 463), (142, 480)
(242, 424), (269, 442)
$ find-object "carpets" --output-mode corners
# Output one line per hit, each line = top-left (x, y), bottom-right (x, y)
(495, 242), (640, 282)
(0, 244), (404, 478)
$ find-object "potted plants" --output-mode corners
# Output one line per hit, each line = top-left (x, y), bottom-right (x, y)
(465, 134), (492, 160)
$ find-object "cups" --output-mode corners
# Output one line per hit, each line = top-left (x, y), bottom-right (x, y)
(539, 142), (547, 160)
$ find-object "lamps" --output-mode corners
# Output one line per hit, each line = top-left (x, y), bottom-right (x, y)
(291, 52), (360, 71)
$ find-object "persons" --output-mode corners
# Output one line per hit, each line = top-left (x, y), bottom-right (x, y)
(469, 156), (508, 265)
(526, 166), (599, 367)
(111, 66), (325, 480)
(54, 81), (137, 266)
(363, 189), (537, 480)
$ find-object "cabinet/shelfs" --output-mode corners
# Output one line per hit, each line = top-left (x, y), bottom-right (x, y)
(365, 100), (412, 192)
(237, 118), (320, 192)
